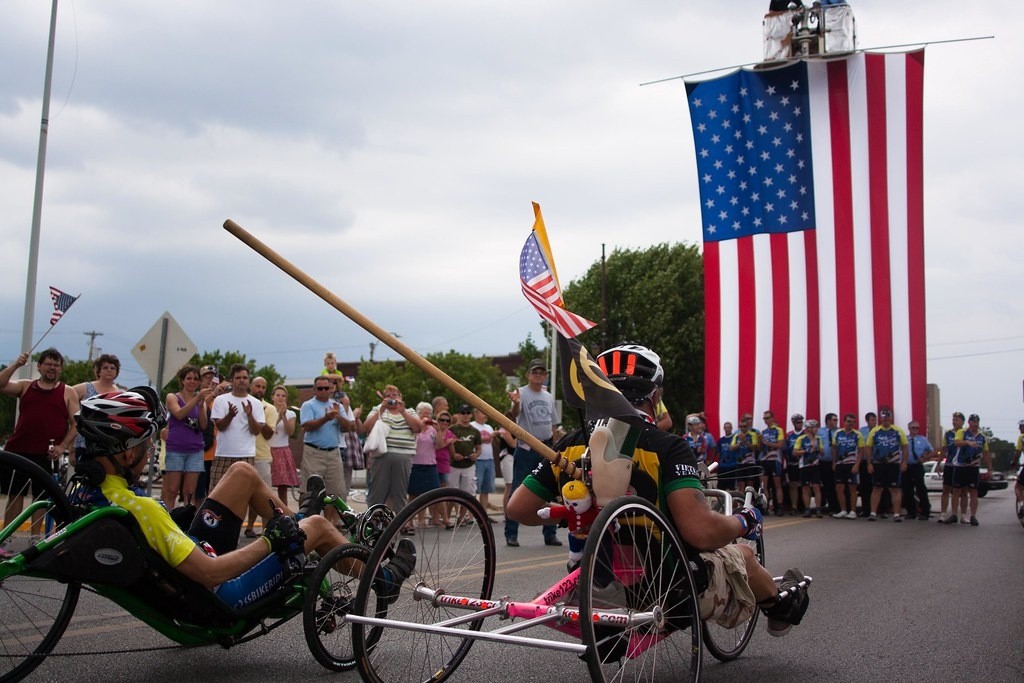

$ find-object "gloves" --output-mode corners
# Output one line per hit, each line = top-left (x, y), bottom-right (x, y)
(262, 515), (297, 554)
(733, 505), (763, 540)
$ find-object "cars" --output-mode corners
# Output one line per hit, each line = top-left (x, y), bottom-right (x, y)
(922, 457), (1008, 498)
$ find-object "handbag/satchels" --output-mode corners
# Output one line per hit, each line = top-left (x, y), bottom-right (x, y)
(362, 411), (391, 459)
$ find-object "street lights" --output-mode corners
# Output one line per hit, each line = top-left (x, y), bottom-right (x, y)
(370, 331), (402, 361)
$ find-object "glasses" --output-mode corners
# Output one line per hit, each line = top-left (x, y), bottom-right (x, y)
(461, 411), (472, 415)
(316, 386), (330, 391)
(438, 418), (450, 423)
(127, 385), (171, 423)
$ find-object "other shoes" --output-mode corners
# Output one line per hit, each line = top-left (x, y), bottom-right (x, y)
(245, 529), (256, 538)
(400, 515), (498, 535)
(1017, 502), (1024, 518)
(2, 536), (13, 552)
(28, 535), (42, 546)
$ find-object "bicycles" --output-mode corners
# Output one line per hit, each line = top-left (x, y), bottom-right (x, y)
(1016, 462), (1024, 528)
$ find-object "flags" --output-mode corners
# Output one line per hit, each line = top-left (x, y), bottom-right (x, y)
(683, 50), (927, 435)
(557, 330), (643, 458)
(211, 367), (219, 384)
(519, 203), (598, 338)
(49, 285), (76, 326)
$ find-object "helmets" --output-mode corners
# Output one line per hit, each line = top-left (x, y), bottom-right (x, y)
(74, 390), (154, 445)
(596, 345), (664, 385)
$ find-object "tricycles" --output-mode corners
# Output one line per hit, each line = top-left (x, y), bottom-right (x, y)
(0, 450), (399, 683)
(347, 447), (812, 683)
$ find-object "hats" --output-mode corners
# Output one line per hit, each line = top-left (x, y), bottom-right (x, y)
(529, 358), (546, 371)
(687, 410), (980, 451)
(201, 365), (216, 375)
(459, 403), (473, 411)
(1019, 419), (1024, 425)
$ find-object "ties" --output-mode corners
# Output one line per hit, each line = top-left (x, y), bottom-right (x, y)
(911, 438), (918, 460)
(828, 430), (832, 448)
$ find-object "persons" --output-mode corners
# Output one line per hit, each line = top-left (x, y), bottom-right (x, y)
(271, 384), (301, 507)
(488, 410), (517, 523)
(363, 385), (498, 536)
(506, 344), (805, 636)
(320, 352), (344, 395)
(69, 354), (125, 488)
(211, 371), (224, 388)
(505, 358), (563, 547)
(161, 365), (208, 510)
(769, 0), (846, 58)
(334, 392), (364, 494)
(299, 376), (351, 532)
(0, 345), (81, 552)
(683, 408), (1024, 525)
(195, 364), (231, 508)
(208, 364), (266, 543)
(68, 390), (417, 604)
(250, 377), (278, 534)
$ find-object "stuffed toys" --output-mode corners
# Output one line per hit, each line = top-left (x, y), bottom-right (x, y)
(537, 481), (598, 571)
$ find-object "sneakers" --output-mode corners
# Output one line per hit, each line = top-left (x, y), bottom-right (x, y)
(298, 474), (326, 516)
(767, 568), (804, 637)
(771, 505), (979, 525)
(374, 538), (417, 603)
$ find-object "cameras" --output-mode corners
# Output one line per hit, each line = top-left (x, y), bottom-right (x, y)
(423, 418), (435, 426)
(184, 415), (199, 430)
(387, 399), (398, 406)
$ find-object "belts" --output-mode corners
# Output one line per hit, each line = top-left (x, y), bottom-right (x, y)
(305, 442), (337, 451)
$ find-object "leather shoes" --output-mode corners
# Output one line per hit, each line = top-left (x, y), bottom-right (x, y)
(545, 536), (562, 547)
(507, 538), (520, 547)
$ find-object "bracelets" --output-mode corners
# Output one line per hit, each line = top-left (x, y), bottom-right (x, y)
(196, 394), (201, 399)
(211, 393), (215, 399)
(70, 450), (75, 454)
